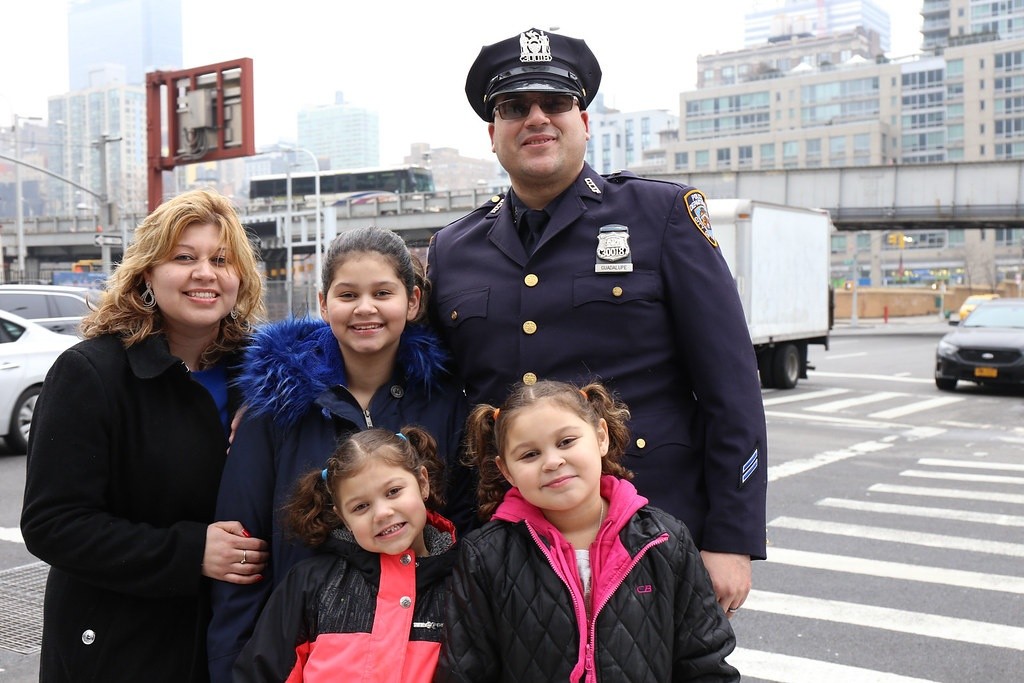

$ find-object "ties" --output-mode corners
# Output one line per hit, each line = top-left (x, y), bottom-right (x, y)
(521, 211), (548, 256)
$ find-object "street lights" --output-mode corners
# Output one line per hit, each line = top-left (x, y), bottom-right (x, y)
(280, 144), (322, 320)
(14, 114), (42, 271)
(285, 164), (303, 317)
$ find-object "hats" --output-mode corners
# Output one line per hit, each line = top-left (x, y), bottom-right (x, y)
(464, 27), (602, 123)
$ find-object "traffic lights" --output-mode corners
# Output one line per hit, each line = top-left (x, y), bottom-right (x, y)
(889, 235), (896, 244)
(844, 281), (853, 291)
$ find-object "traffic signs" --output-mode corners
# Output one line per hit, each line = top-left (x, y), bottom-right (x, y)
(95, 235), (123, 246)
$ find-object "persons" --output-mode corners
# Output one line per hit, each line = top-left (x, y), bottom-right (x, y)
(439, 380), (739, 683)
(19, 187), (264, 683)
(206, 224), (469, 683)
(232, 426), (456, 683)
(421, 27), (767, 618)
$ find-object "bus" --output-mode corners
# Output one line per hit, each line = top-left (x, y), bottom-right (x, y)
(248, 164), (440, 221)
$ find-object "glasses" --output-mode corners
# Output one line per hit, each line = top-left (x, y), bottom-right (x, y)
(491, 92), (581, 123)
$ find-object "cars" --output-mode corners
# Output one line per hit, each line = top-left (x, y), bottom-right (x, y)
(959, 294), (1000, 319)
(935, 299), (1024, 396)
(0, 283), (105, 339)
(0, 309), (87, 455)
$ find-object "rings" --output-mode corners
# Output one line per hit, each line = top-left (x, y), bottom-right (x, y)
(728, 607), (738, 613)
(241, 549), (246, 563)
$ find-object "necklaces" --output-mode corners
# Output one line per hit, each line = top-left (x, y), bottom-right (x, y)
(598, 498), (603, 528)
